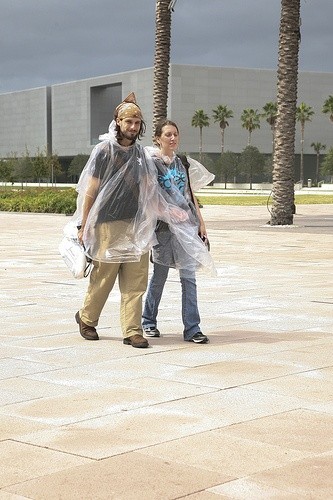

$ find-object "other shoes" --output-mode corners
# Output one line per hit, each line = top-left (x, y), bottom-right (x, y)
(74, 311), (99, 340)
(123, 333), (149, 347)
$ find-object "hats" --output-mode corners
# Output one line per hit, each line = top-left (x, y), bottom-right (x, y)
(113, 92), (143, 120)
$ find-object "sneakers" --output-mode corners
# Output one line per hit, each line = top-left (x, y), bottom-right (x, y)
(189, 331), (209, 343)
(143, 327), (160, 337)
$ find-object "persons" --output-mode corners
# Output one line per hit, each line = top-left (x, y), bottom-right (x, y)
(140, 119), (211, 344)
(75, 100), (161, 348)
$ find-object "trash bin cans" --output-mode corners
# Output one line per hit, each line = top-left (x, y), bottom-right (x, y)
(308, 179), (312, 188)
